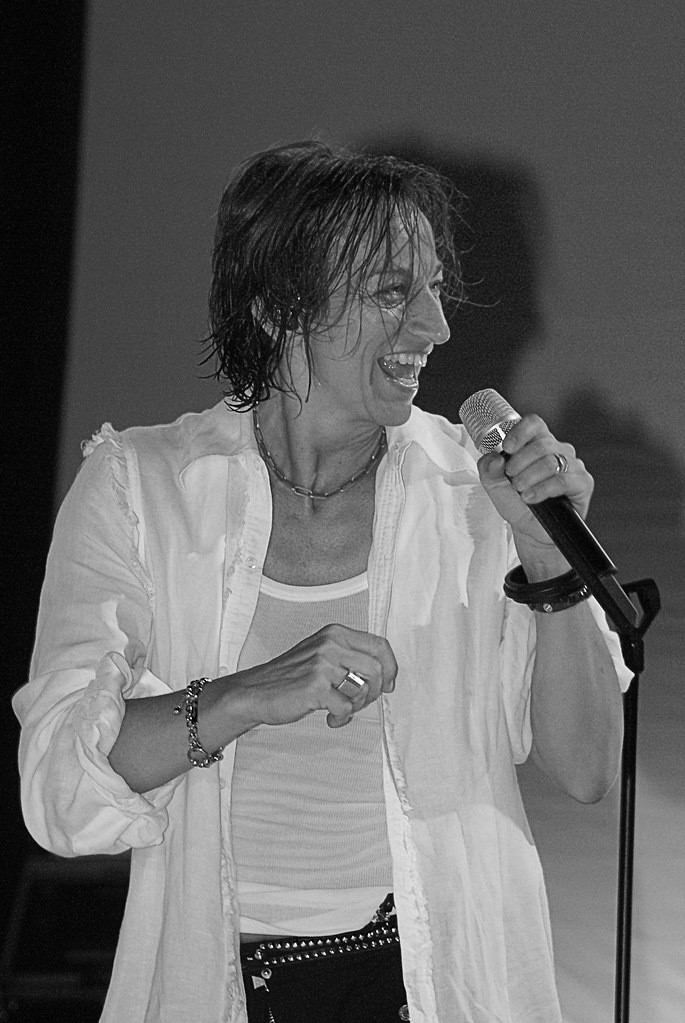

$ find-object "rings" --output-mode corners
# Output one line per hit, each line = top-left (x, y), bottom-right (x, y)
(336, 672), (365, 699)
(554, 452), (569, 473)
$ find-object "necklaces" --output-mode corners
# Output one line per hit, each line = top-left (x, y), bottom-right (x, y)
(248, 390), (388, 500)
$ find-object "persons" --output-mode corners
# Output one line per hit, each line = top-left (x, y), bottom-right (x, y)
(10, 131), (634, 1023)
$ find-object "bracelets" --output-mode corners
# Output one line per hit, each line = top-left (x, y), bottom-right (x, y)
(173, 679), (224, 768)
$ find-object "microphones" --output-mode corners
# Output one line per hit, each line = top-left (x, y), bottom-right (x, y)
(459, 388), (640, 636)
